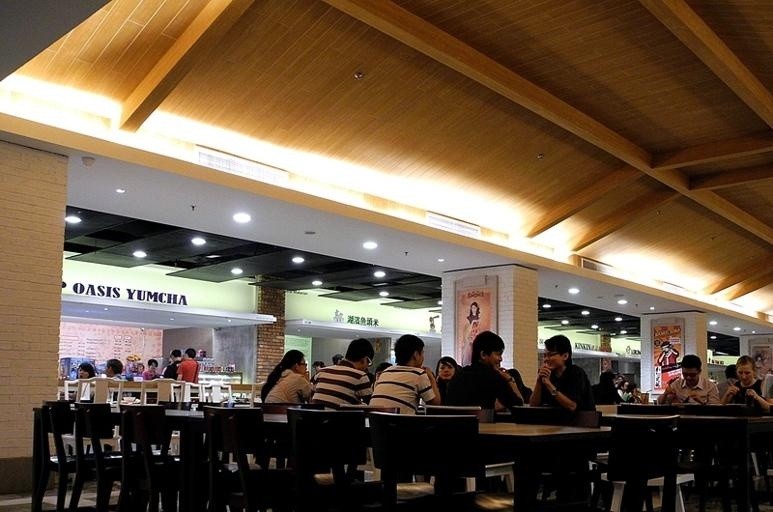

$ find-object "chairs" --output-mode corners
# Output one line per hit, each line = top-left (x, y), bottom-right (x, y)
(31, 374), (773, 511)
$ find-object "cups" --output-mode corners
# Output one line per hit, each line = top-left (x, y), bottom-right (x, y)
(640, 393), (649, 404)
(743, 393), (753, 407)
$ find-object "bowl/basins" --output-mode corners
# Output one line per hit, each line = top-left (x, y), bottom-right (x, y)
(122, 396), (136, 403)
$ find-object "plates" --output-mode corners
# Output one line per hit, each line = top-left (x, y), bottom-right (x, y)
(118, 400), (140, 405)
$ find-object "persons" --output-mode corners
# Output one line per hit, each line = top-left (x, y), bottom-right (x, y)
(69, 349), (207, 397)
(465, 301), (479, 325)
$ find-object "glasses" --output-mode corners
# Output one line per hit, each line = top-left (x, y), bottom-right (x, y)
(367, 356), (373, 367)
(297, 363), (307, 366)
(682, 370), (700, 377)
